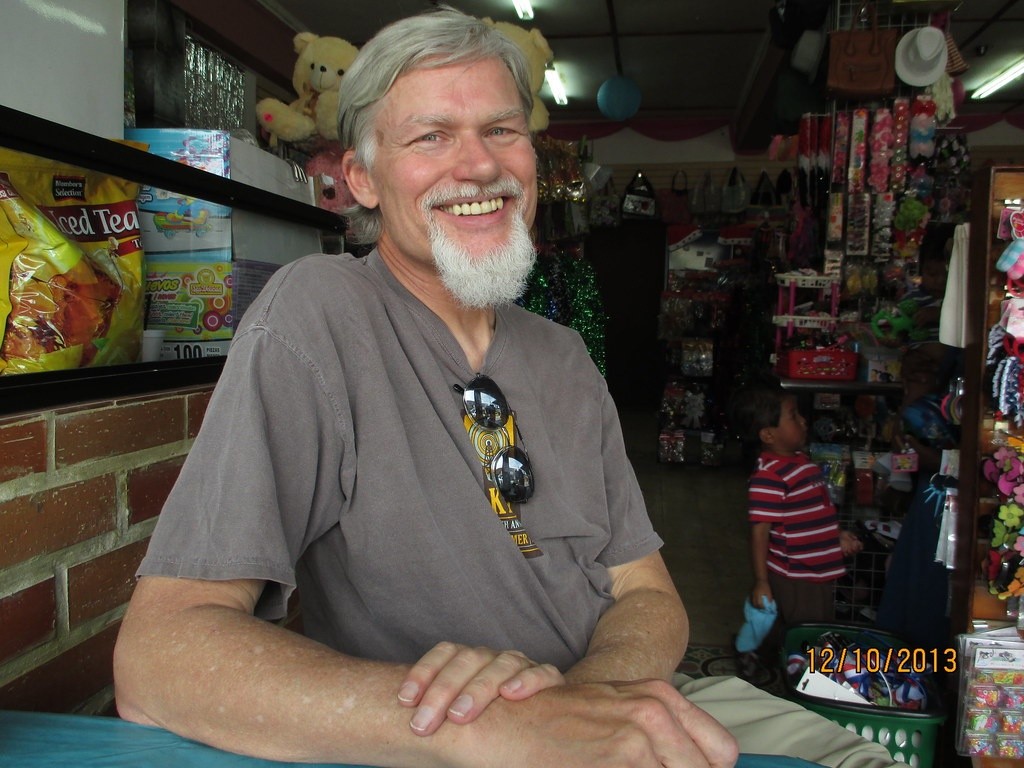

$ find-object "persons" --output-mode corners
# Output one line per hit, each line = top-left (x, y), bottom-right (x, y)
(873, 224), (953, 684)
(112, 4), (911, 768)
(746, 392), (865, 629)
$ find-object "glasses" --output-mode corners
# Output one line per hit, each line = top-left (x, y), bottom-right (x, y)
(454, 373), (535, 505)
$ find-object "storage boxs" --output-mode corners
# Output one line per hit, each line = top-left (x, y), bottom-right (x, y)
(124, 126), (325, 361)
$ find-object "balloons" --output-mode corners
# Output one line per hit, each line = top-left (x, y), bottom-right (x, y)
(597, 74), (641, 120)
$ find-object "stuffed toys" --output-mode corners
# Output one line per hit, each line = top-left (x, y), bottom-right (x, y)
(303, 141), (359, 233)
(481, 16), (554, 133)
(256, 31), (359, 143)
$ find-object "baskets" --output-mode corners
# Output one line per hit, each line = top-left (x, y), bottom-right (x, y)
(783, 623), (947, 768)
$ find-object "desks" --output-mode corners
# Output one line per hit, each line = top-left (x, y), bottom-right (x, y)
(0, 708), (827, 768)
(777, 374), (901, 441)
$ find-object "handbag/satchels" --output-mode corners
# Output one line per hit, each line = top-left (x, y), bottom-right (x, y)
(590, 168), (792, 228)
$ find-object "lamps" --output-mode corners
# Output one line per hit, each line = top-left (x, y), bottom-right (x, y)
(512, 0), (534, 20)
(544, 61), (569, 105)
